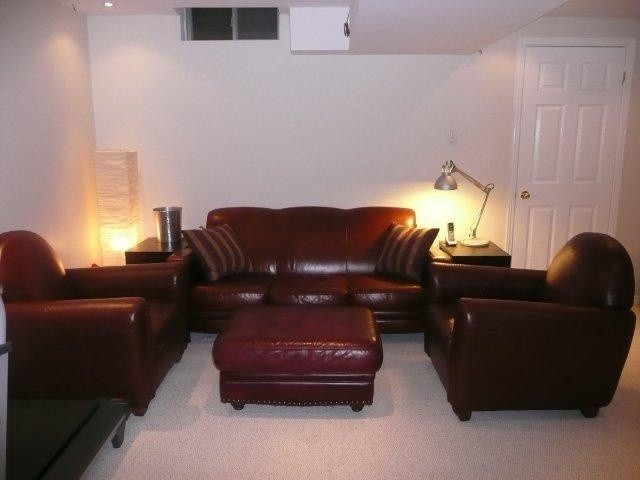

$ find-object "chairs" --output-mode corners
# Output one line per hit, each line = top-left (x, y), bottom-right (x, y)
(0, 229), (193, 417)
(423, 232), (637, 422)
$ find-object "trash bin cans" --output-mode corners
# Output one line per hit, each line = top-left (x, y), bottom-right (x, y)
(153, 206), (183, 244)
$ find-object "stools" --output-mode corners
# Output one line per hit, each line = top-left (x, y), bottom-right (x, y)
(212, 304), (384, 414)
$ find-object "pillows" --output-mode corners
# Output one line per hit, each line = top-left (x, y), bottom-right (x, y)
(168, 203), (453, 334)
(180, 222), (254, 284)
(375, 219), (439, 284)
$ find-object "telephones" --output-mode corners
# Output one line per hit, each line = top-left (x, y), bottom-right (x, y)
(444, 222), (458, 247)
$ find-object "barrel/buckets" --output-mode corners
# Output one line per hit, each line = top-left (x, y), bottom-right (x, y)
(153, 205), (183, 249)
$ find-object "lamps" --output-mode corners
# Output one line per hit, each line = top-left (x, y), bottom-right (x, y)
(433, 160), (496, 248)
